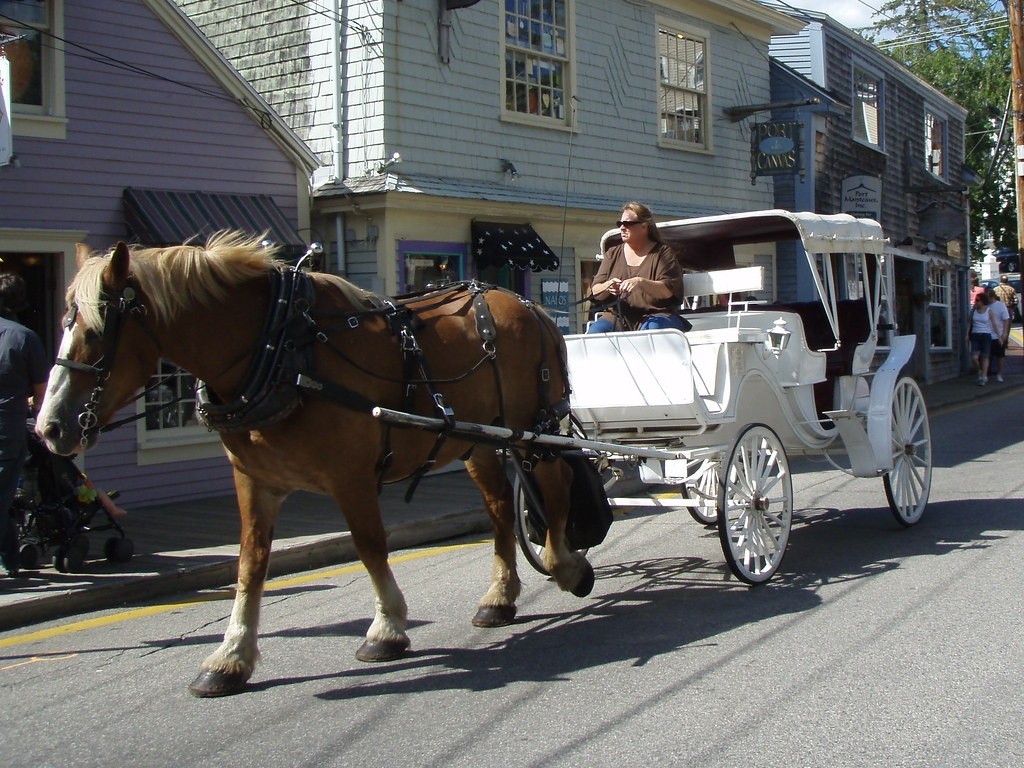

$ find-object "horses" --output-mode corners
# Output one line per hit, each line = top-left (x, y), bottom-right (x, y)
(33, 223), (594, 700)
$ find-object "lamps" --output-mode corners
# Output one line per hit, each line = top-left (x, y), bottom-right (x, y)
(504, 160), (522, 181)
(766, 316), (791, 359)
(921, 242), (937, 255)
(893, 236), (914, 252)
(375, 151), (404, 174)
(440, 258), (449, 270)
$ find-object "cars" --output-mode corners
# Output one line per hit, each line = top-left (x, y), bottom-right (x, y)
(976, 274), (1024, 324)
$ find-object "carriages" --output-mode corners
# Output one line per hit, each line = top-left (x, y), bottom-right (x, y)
(34, 207), (934, 699)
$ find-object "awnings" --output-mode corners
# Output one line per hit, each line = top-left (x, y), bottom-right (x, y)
(122, 186), (308, 263)
(471, 221), (559, 272)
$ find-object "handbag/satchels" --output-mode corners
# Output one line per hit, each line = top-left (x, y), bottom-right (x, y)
(1008, 304), (1022, 323)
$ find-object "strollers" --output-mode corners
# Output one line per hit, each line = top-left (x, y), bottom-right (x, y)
(19, 404), (136, 573)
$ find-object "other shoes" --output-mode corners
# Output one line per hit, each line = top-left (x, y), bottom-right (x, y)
(996, 374), (1004, 381)
(978, 377), (988, 385)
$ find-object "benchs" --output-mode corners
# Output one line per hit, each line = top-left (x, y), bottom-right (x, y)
(680, 310), (828, 387)
(682, 298), (884, 375)
(680, 266), (768, 344)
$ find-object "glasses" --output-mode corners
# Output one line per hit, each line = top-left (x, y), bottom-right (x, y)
(616, 221), (646, 228)
(976, 299), (983, 301)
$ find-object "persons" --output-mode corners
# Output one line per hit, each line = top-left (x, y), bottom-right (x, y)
(587, 202), (693, 335)
(965, 270), (1021, 386)
(1, 263), (128, 578)
